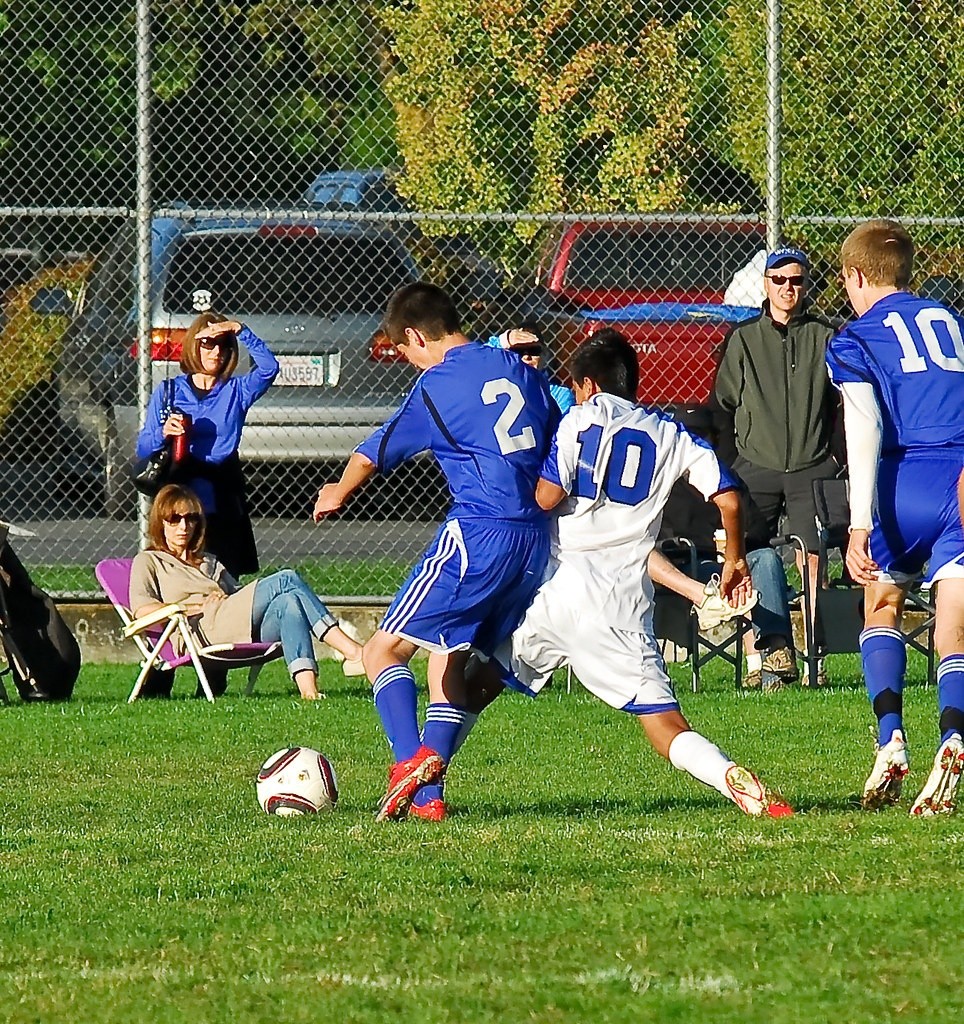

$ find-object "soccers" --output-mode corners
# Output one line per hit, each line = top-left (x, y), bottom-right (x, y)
(255, 746), (338, 816)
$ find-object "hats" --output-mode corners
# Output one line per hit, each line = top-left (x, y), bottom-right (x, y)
(765, 249), (808, 268)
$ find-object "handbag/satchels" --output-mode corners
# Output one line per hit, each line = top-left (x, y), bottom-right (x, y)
(130, 378), (175, 498)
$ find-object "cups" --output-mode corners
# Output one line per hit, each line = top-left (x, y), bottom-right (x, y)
(172, 413), (192, 465)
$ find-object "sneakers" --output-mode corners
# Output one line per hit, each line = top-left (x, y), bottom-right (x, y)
(724, 765), (794, 818)
(763, 646), (798, 682)
(909, 732), (964, 815)
(693, 573), (761, 630)
(409, 799), (444, 822)
(864, 728), (907, 808)
(376, 744), (444, 822)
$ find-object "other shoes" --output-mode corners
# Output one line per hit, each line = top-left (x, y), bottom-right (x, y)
(803, 674), (826, 686)
(743, 671), (760, 688)
(341, 659), (367, 676)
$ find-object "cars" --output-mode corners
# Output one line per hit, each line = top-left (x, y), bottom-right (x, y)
(305, 169), (419, 214)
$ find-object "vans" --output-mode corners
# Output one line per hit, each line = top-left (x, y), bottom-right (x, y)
(506, 222), (769, 422)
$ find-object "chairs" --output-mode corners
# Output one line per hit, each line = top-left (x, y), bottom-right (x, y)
(813, 479), (936, 686)
(655, 533), (813, 693)
(95, 559), (285, 703)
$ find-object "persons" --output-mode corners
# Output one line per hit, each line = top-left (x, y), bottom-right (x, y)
(137, 311), (279, 700)
(128, 485), (366, 700)
(653, 404), (799, 688)
(714, 249), (829, 689)
(448, 332), (792, 817)
(823, 219), (964, 816)
(313, 281), (561, 821)
(482, 321), (574, 416)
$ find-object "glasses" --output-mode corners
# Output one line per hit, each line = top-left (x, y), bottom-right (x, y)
(160, 513), (199, 526)
(200, 337), (231, 351)
(766, 275), (806, 286)
(510, 346), (543, 356)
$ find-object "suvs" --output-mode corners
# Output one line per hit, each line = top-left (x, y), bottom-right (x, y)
(27, 201), (434, 519)
